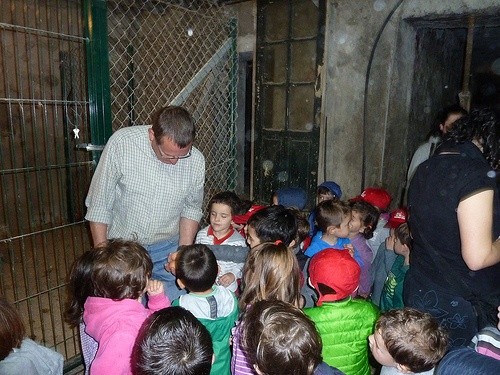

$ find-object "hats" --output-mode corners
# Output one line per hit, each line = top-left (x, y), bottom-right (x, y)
(309, 249), (361, 306)
(383, 208), (408, 229)
(348, 186), (390, 211)
(321, 181), (342, 200)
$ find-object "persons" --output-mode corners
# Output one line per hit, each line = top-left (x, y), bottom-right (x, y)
(85, 106), (207, 305)
(401, 101), (500, 351)
(0, 296), (63, 375)
(63, 181), (500, 375)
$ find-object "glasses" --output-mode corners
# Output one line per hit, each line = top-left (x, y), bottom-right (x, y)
(159, 141), (193, 159)
